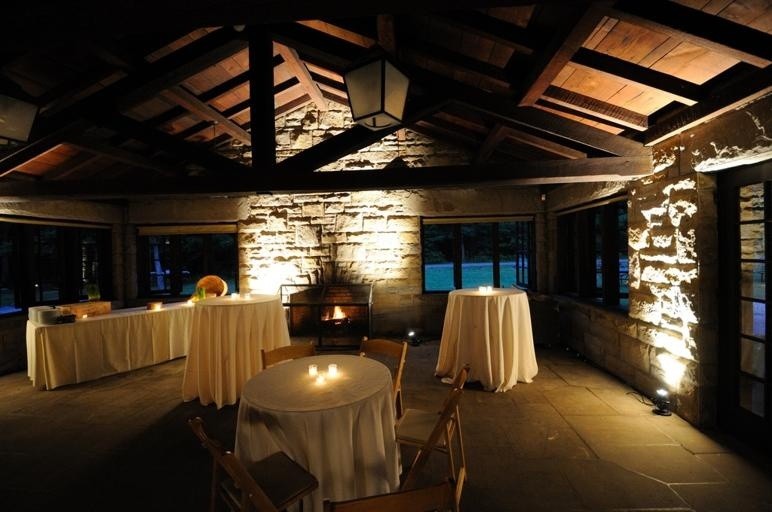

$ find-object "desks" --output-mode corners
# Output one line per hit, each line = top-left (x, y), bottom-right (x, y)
(27, 301), (196, 390)
(435, 288), (539, 396)
(233, 352), (404, 505)
(182, 295), (290, 410)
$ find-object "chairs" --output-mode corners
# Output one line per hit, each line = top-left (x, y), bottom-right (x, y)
(188, 414), (320, 511)
(396, 368), (469, 491)
(259, 345), (301, 369)
(356, 337), (409, 419)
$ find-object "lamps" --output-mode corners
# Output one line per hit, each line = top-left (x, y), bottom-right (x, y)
(342, 36), (412, 133)
(652, 389), (672, 416)
(0, 94), (40, 148)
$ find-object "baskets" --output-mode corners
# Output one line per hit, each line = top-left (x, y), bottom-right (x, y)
(57, 302), (111, 319)
